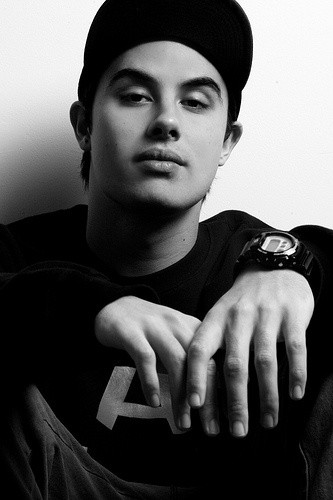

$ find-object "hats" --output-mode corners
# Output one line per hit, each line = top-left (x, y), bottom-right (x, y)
(79, 1), (253, 121)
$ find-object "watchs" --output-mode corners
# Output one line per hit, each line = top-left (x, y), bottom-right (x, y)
(234, 227), (323, 299)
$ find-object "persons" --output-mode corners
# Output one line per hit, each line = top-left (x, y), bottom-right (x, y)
(0, 0), (333, 499)
